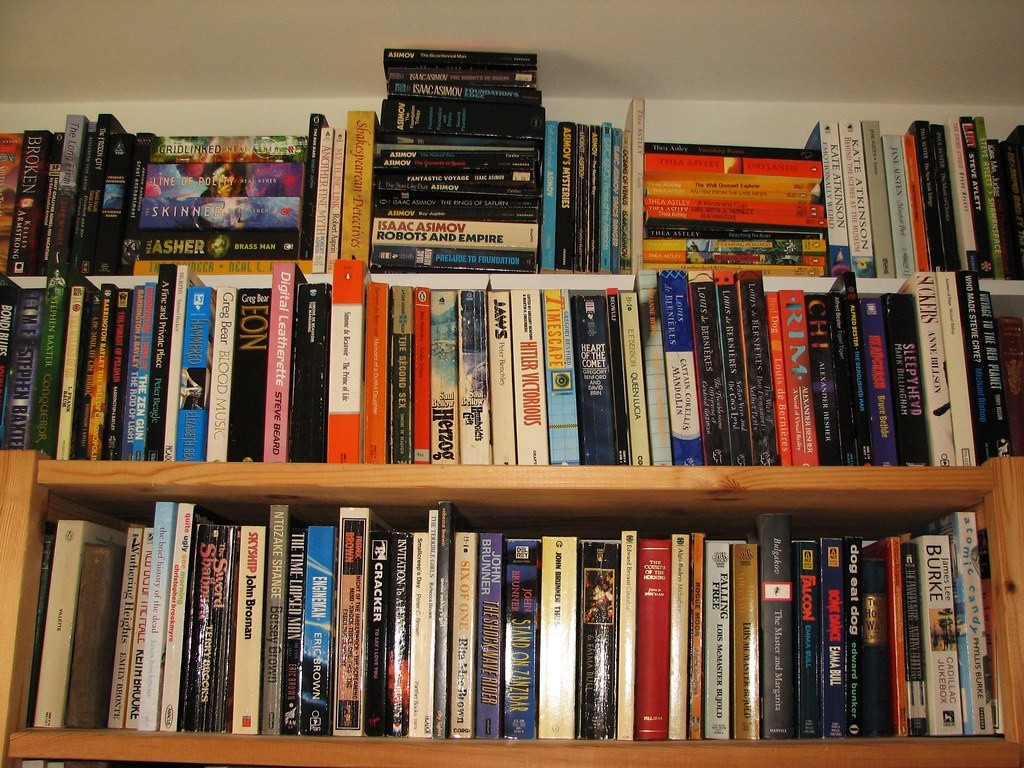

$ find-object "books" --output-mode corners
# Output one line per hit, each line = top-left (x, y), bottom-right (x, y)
(0, 48), (1024, 274)
(0, 259), (1024, 475)
(15, 490), (1005, 768)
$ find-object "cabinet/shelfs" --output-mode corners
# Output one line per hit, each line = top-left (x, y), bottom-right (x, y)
(0, 271), (1023, 768)
